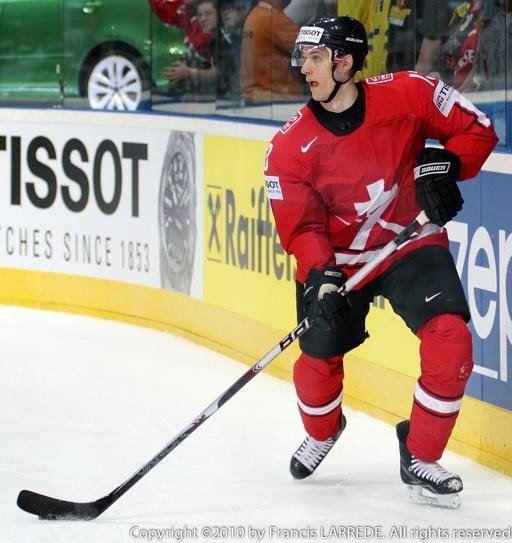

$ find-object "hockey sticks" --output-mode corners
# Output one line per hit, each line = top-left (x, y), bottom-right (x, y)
(18, 210), (430, 521)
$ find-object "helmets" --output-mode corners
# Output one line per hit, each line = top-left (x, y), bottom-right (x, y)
(290, 16), (368, 70)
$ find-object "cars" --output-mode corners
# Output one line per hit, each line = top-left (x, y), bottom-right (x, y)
(0, 0), (192, 112)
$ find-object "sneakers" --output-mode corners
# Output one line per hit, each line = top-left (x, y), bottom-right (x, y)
(397, 421), (463, 495)
(290, 414), (346, 478)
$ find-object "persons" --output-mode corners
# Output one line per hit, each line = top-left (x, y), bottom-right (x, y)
(259, 14), (501, 496)
(147, 0), (512, 103)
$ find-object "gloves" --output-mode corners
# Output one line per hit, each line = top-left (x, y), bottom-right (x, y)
(302, 269), (351, 332)
(415, 148), (463, 226)
